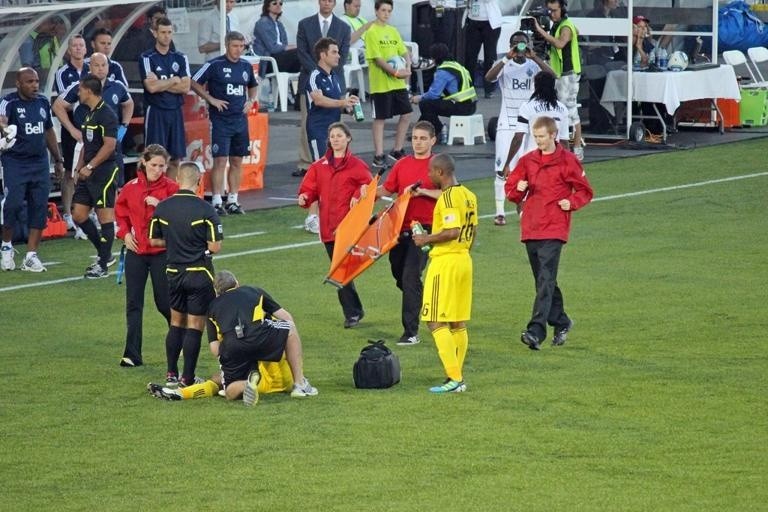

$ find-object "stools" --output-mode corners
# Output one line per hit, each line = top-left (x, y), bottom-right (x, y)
(446, 112), (487, 149)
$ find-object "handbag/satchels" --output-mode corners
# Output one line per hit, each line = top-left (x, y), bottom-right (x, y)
(353, 339), (401, 389)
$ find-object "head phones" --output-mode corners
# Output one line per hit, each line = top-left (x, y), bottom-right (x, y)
(562, 0), (569, 19)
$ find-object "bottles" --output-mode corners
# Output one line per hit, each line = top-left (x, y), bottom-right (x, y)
(352, 102), (364, 122)
(247, 100), (259, 117)
(410, 219), (433, 253)
(198, 101), (210, 119)
(632, 46), (668, 71)
(517, 43), (527, 54)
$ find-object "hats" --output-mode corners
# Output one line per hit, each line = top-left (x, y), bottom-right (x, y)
(633, 15), (650, 25)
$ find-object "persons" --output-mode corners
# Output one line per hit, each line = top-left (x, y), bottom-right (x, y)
(253, 0), (414, 177)
(146, 317), (294, 401)
(410, 154), (477, 395)
(205, 268), (320, 407)
(114, 144), (178, 367)
(1, 4), (258, 270)
(588, 1), (679, 138)
(359, 119), (438, 347)
(148, 162), (223, 385)
(502, 117), (594, 350)
(410, 1), (584, 225)
(297, 120), (372, 329)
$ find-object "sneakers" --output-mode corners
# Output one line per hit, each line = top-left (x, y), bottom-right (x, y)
(84, 252), (116, 280)
(397, 335), (420, 345)
(212, 202), (245, 217)
(574, 147), (584, 161)
(290, 377), (318, 397)
(516, 203), (524, 218)
(64, 212), (120, 240)
(552, 319), (573, 346)
(521, 332), (540, 351)
(243, 370), (262, 409)
(147, 371), (205, 401)
(1, 241), (15, 271)
(293, 166), (307, 176)
(20, 251), (47, 272)
(120, 357), (135, 367)
(344, 309), (364, 328)
(304, 215), (320, 233)
(485, 90), (495, 98)
(429, 377), (467, 393)
(372, 149), (407, 168)
(494, 215), (505, 225)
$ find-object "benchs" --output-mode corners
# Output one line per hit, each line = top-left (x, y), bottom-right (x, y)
(0, 121), (144, 222)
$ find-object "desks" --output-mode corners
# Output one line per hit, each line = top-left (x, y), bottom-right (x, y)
(599, 55), (740, 146)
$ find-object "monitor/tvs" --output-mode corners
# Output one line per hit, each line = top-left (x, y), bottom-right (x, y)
(690, 36), (704, 63)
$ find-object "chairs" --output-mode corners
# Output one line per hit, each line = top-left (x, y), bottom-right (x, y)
(722, 47), (761, 92)
(604, 59), (626, 74)
(746, 43), (767, 91)
(245, 36), (426, 114)
(579, 62), (624, 138)
